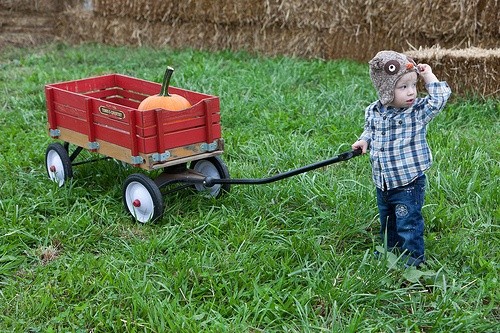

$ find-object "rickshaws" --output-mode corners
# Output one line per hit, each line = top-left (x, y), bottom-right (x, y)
(44, 74), (368, 224)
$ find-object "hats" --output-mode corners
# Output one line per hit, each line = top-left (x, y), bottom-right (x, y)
(369, 50), (421, 105)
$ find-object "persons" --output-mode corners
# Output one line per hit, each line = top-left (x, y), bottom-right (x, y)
(351, 50), (451, 292)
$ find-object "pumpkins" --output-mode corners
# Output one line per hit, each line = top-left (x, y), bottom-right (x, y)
(138, 66), (191, 111)
